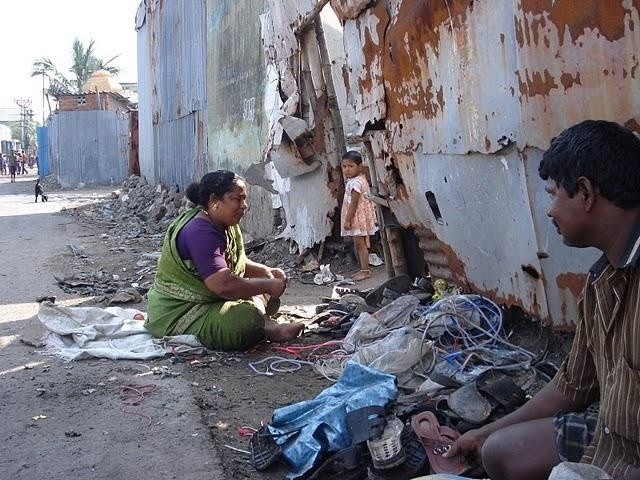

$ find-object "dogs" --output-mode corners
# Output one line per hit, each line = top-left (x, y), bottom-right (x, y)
(34, 179), (48, 202)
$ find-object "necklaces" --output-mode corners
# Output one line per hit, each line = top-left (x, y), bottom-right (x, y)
(201, 209), (217, 225)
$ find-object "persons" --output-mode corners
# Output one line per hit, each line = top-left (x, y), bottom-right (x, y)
(143, 169), (305, 352)
(0, 150), (39, 183)
(442, 120), (640, 480)
(340, 150), (379, 281)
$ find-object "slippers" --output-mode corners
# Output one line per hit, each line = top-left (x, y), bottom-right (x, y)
(309, 370), (526, 479)
(299, 270), (316, 284)
(250, 425), (283, 472)
(299, 279), (451, 337)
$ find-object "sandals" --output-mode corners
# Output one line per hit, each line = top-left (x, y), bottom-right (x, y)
(299, 259), (319, 272)
